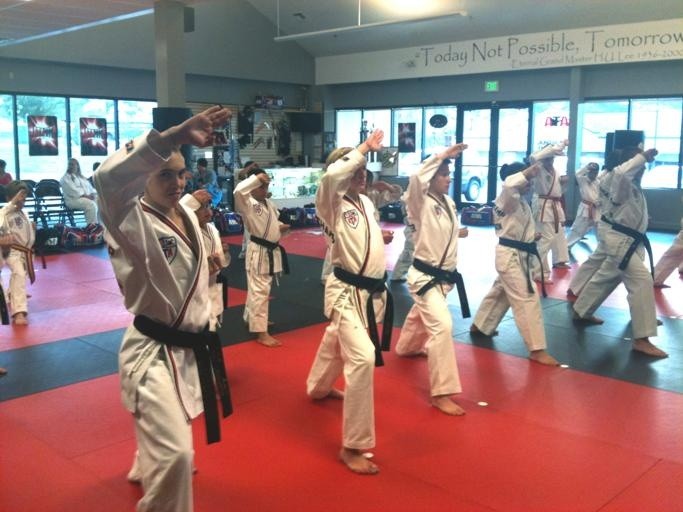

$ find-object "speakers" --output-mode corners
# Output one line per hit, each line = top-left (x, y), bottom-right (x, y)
(184, 8), (195, 32)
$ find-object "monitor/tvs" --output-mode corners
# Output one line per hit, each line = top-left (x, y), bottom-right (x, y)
(285, 112), (323, 133)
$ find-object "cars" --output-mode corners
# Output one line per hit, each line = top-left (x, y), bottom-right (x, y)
(399, 159), (484, 201)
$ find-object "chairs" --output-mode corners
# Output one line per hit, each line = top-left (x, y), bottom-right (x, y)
(0, 178), (95, 228)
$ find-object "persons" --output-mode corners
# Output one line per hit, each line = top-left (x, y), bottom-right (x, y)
(566, 150), (621, 302)
(232, 168), (291, 347)
(192, 157), (223, 209)
(319, 169), (403, 286)
(394, 142), (470, 417)
(88, 162), (102, 188)
(529, 139), (572, 285)
(390, 181), (431, 283)
(471, 161), (560, 365)
(179, 189), (231, 401)
(651, 230), (682, 288)
(59, 158), (103, 235)
(92, 105), (233, 511)
(572, 146), (670, 358)
(1, 180), (37, 326)
(303, 127), (395, 475)
(0, 158), (12, 185)
(232, 160), (259, 260)
(564, 161), (601, 251)
(1, 284), (9, 374)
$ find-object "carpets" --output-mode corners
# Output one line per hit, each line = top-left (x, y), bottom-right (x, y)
(1, 249), (273, 351)
(0, 313), (683, 512)
(219, 219), (500, 271)
(534, 242), (683, 320)
